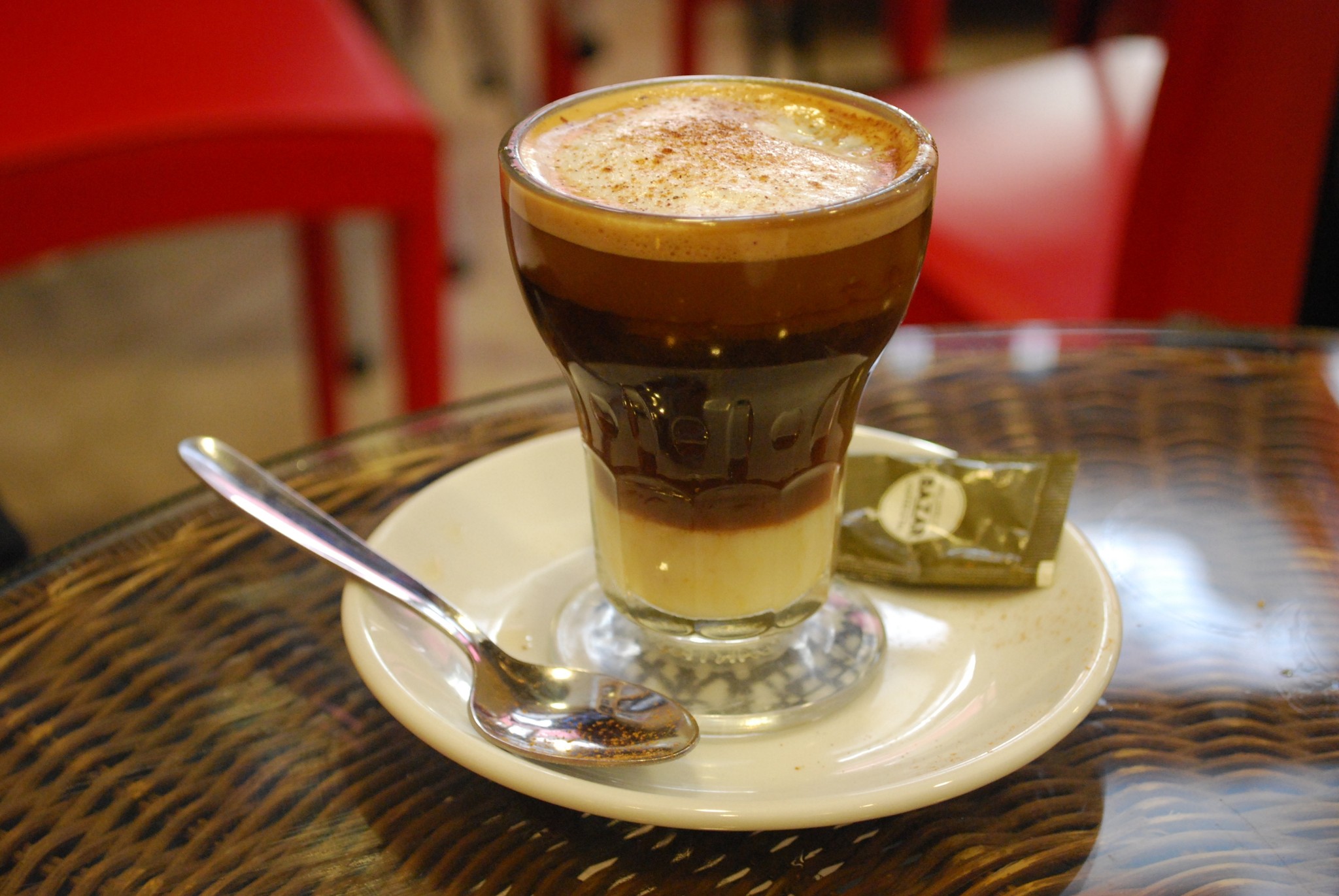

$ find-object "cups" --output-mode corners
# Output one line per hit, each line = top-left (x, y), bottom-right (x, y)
(497, 75), (941, 736)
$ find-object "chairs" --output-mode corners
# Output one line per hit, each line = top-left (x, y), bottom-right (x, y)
(874, 0), (1339, 335)
(0, 0), (447, 448)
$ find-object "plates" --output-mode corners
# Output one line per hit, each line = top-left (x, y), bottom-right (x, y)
(339, 423), (1123, 833)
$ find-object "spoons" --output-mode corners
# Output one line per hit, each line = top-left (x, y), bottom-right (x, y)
(175, 434), (701, 766)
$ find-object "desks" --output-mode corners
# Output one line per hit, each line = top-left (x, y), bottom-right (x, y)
(0, 334), (1339, 896)
